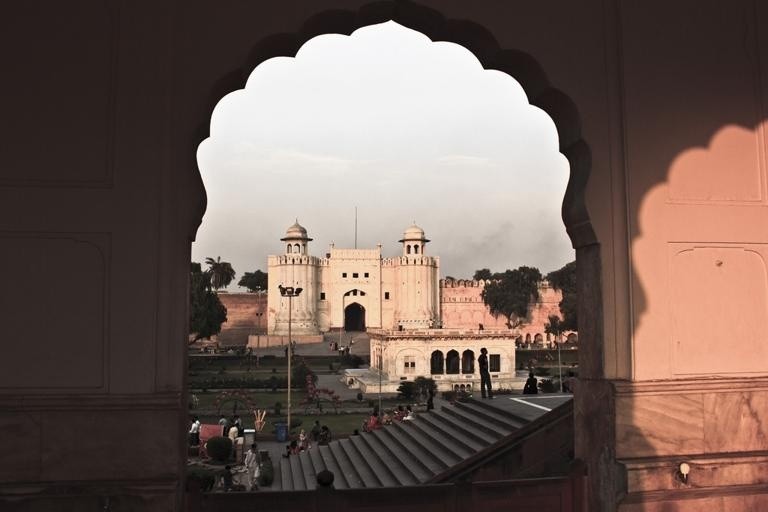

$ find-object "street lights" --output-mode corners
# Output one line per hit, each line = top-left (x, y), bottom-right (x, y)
(277, 284), (302, 435)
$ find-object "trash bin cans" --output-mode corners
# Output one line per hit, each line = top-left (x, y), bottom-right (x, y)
(244, 429), (256, 448)
(330, 342), (337, 351)
(274, 422), (287, 442)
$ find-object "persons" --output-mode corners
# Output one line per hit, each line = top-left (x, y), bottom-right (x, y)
(429, 319), (434, 329)
(478, 348), (497, 399)
(562, 372), (576, 393)
(188, 405), (415, 491)
(524, 373), (538, 394)
(330, 341), (350, 357)
